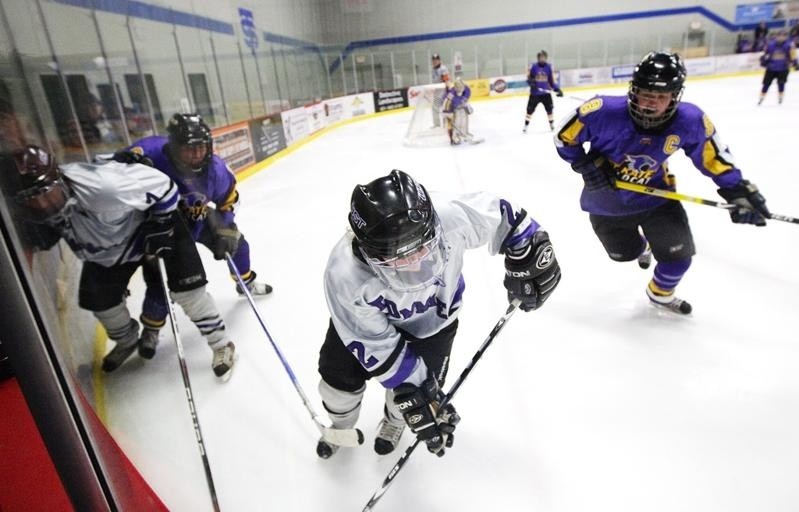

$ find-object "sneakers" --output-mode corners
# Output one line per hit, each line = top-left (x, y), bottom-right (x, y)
(450, 140), (454, 143)
(430, 125), (440, 129)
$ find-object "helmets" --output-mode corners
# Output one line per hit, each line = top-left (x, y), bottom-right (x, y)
(775, 30), (787, 47)
(628, 50), (686, 129)
(537, 50), (548, 67)
(348, 169), (448, 293)
(166, 112), (213, 179)
(454, 77), (465, 97)
(0, 144), (77, 229)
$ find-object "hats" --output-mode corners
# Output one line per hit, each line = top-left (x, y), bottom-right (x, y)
(432, 53), (439, 59)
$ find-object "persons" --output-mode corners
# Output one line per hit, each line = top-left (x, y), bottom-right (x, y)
(554, 47), (768, 315)
(441, 77), (471, 147)
(521, 51), (563, 130)
(735, 20), (799, 105)
(315, 169), (561, 460)
(0, 145), (234, 382)
(116, 112), (272, 359)
(429, 53), (449, 129)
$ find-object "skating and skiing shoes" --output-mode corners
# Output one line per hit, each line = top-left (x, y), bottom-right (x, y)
(237, 282), (273, 296)
(374, 417), (405, 455)
(101, 338), (140, 374)
(212, 342), (237, 382)
(649, 298), (694, 318)
(138, 327), (159, 367)
(550, 122), (554, 131)
(522, 125), (527, 131)
(638, 235), (652, 269)
(316, 425), (340, 459)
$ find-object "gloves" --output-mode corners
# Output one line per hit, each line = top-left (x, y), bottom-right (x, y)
(790, 60), (799, 72)
(571, 150), (617, 194)
(553, 88), (564, 98)
(529, 82), (539, 92)
(393, 368), (460, 457)
(759, 56), (771, 69)
(504, 231), (561, 312)
(717, 180), (771, 227)
(214, 222), (244, 260)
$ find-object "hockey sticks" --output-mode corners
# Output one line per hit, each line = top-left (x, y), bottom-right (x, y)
(424, 96), (483, 145)
(226, 251), (365, 446)
